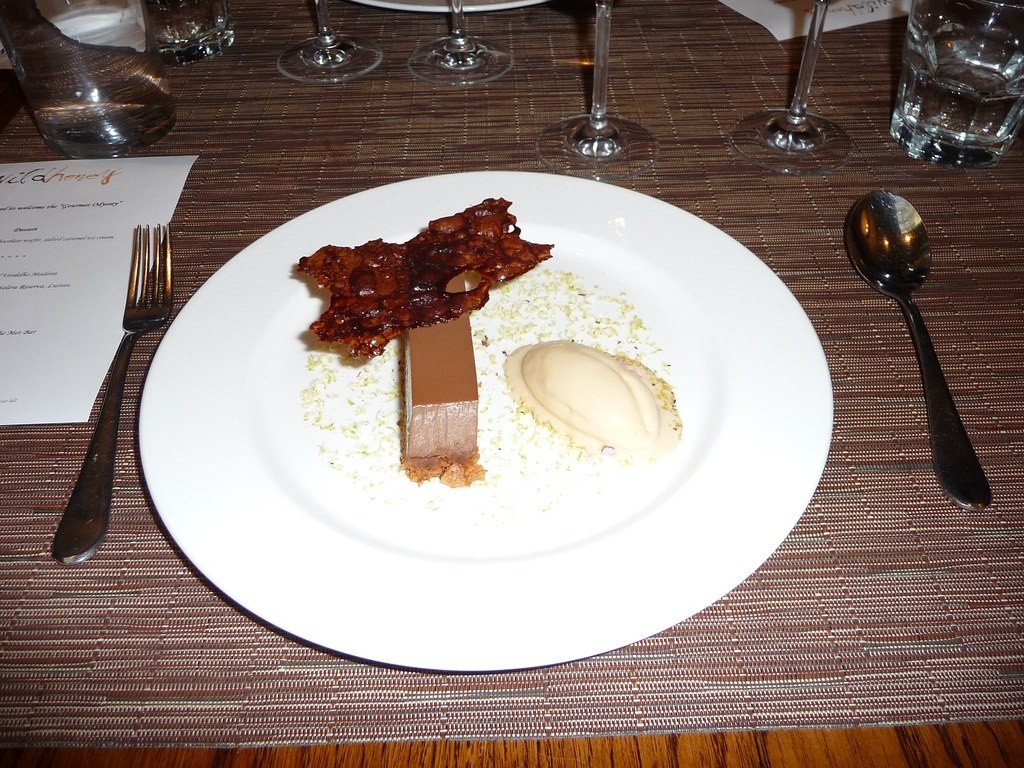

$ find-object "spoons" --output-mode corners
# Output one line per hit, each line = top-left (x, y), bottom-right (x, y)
(846, 190), (991, 511)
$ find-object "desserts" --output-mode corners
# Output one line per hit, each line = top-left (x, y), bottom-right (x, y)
(503, 341), (682, 469)
(296, 195), (555, 488)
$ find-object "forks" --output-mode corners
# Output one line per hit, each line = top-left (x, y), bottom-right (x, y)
(52, 223), (172, 566)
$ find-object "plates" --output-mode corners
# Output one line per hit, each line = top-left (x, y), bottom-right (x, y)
(139, 171), (833, 671)
(350, 0), (549, 12)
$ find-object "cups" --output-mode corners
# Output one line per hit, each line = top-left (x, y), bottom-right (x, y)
(890, 0), (1024, 170)
(143, 0), (234, 65)
(0, 0), (176, 158)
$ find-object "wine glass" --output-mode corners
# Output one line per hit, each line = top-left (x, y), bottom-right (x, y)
(730, 0), (855, 175)
(535, 0), (662, 181)
(406, 0), (515, 85)
(277, 0), (383, 82)
